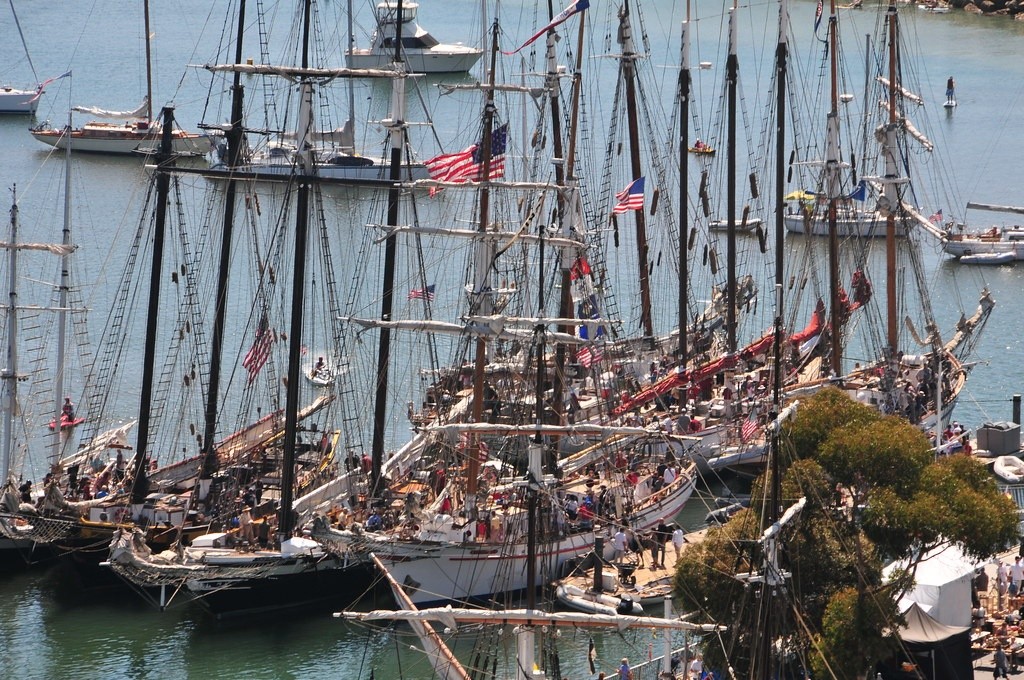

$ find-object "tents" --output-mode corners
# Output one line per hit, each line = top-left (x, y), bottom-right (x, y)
(875, 602), (975, 680)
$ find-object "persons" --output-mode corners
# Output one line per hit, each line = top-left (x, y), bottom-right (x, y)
(971, 538), (1024, 680)
(945, 76), (953, 104)
(16, 331), (971, 570)
(989, 226), (997, 237)
(898, 662), (918, 680)
(598, 643), (703, 680)
(695, 139), (702, 147)
(797, 201), (806, 214)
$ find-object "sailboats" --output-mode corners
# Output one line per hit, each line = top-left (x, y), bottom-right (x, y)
(0, 0), (1024, 680)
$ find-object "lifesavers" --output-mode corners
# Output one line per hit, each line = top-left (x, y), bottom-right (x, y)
(662, 355), (667, 365)
(115, 509), (129, 524)
(484, 472), (495, 481)
(499, 533), (503, 541)
(873, 369), (881, 377)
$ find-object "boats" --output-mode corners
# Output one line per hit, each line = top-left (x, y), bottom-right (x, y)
(345, 0), (485, 72)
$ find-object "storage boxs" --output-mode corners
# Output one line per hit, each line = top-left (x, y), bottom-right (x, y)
(281, 537), (321, 555)
(192, 533), (227, 549)
(603, 572), (617, 591)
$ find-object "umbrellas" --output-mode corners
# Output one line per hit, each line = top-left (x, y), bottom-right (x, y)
(784, 190), (817, 200)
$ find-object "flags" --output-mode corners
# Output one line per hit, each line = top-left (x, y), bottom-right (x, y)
(408, 284), (435, 302)
(423, 122), (508, 199)
(614, 176), (645, 213)
(740, 401), (759, 442)
(570, 258), (603, 339)
(588, 637), (597, 675)
(929, 209), (943, 224)
(455, 422), (488, 460)
(242, 311), (273, 381)
(576, 344), (605, 367)
